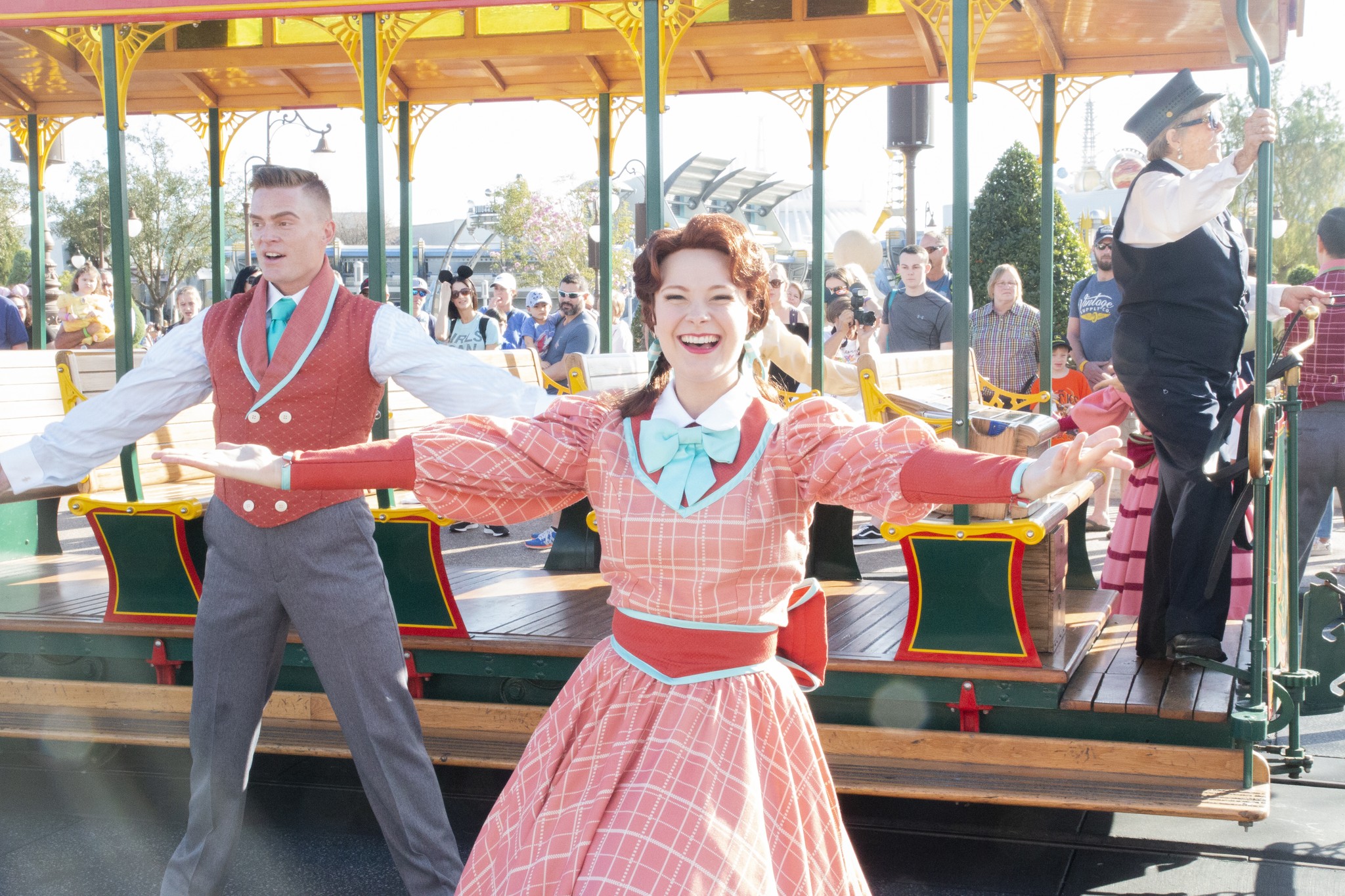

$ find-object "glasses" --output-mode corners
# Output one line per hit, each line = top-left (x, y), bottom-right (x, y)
(17, 304), (26, 310)
(1096, 243), (1112, 250)
(413, 290), (427, 297)
(246, 276), (255, 284)
(925, 244), (945, 253)
(558, 290), (587, 298)
(996, 281), (1017, 288)
(1167, 109), (1223, 145)
(769, 279), (784, 288)
(453, 287), (471, 299)
(830, 285), (847, 291)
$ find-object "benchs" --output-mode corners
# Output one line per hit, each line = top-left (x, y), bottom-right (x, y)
(0, 349), (217, 626)
(363, 346), (571, 639)
(856, 349), (1106, 667)
(566, 351), (821, 533)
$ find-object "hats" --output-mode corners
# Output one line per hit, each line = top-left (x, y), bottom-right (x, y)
(1093, 225), (1115, 246)
(525, 288), (551, 307)
(413, 278), (431, 295)
(1124, 68), (1225, 146)
(490, 272), (516, 292)
(1052, 334), (1072, 353)
(359, 277), (388, 295)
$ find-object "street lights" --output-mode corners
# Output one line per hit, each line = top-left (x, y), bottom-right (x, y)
(98, 188), (142, 268)
(925, 201), (936, 229)
(1242, 190), (1288, 249)
(265, 110), (336, 165)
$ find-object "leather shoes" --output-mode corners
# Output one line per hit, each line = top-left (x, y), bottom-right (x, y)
(1135, 635), (1226, 665)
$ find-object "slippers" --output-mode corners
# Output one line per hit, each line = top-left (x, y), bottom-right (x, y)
(1086, 519), (1111, 540)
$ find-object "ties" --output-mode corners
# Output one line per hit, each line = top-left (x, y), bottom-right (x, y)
(267, 299), (297, 363)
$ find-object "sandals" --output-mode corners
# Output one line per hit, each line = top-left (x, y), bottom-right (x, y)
(1330, 565), (1345, 574)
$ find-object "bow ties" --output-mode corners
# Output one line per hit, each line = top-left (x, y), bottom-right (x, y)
(639, 420), (741, 506)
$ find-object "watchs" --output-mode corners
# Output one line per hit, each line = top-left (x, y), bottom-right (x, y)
(81, 345), (87, 350)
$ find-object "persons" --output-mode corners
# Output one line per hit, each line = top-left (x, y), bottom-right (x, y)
(55, 261), (146, 350)
(1282, 207), (1345, 582)
(0, 295), (28, 350)
(597, 289), (633, 353)
(1110, 66), (1336, 664)
(435, 265), (510, 538)
(525, 273), (600, 550)
(230, 265), (262, 298)
(397, 278), (437, 341)
(485, 307), (515, 349)
(755, 224), (1141, 532)
(479, 273), (530, 349)
(521, 288), (565, 368)
(165, 286), (202, 334)
(151, 213), (1134, 896)
(359, 277), (393, 306)
(0, 168), (551, 896)
(142, 320), (168, 348)
(0, 284), (56, 350)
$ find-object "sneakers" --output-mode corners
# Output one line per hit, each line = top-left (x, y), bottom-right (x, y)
(484, 523), (510, 537)
(524, 526), (558, 549)
(1311, 537), (1330, 556)
(851, 524), (886, 545)
(449, 521), (479, 532)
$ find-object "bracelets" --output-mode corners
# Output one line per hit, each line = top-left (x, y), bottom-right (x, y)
(83, 328), (90, 338)
(65, 313), (69, 320)
